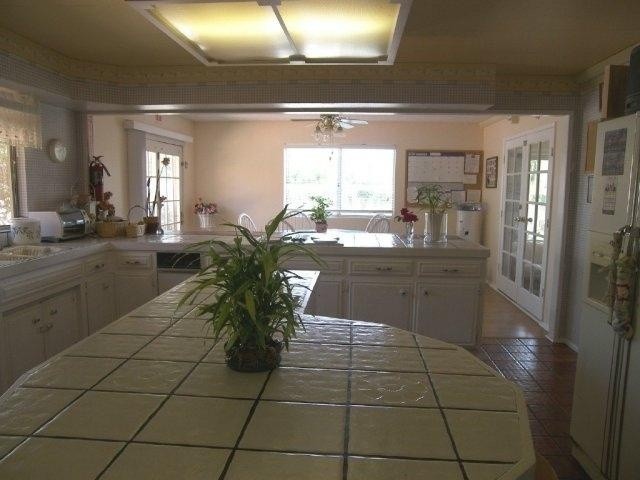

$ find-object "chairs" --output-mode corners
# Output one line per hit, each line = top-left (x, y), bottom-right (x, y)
(236, 210), (312, 232)
(364, 215), (391, 233)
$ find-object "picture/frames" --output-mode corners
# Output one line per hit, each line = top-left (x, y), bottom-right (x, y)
(486, 157), (497, 187)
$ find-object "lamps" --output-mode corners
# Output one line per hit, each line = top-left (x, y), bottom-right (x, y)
(312, 114), (347, 161)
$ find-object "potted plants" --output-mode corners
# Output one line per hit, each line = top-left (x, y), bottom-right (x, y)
(309, 194), (330, 232)
(413, 181), (454, 246)
(172, 204), (327, 378)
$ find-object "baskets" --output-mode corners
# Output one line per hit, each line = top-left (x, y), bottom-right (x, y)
(125, 206), (147, 238)
(94, 219), (124, 238)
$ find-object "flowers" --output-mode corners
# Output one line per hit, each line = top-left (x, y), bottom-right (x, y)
(192, 199), (218, 214)
(394, 208), (418, 235)
(95, 155), (171, 224)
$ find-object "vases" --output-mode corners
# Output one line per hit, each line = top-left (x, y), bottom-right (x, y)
(96, 216), (158, 239)
(405, 223), (415, 244)
(199, 214), (216, 228)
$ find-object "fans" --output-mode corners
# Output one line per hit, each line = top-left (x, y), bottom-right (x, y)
(303, 112), (369, 131)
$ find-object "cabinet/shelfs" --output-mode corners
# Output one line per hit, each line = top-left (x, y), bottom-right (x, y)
(0, 251), (157, 397)
(347, 256), (486, 350)
